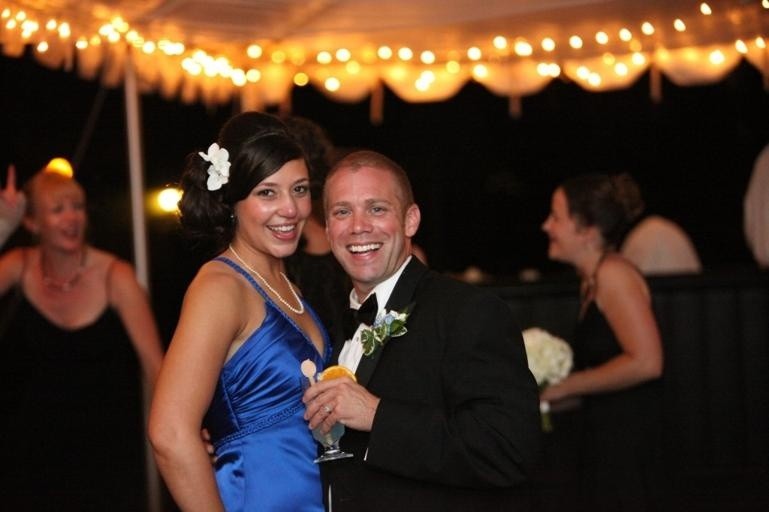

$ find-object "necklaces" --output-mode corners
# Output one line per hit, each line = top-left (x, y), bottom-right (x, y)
(577, 250), (608, 302)
(227, 241), (305, 315)
(37, 242), (86, 291)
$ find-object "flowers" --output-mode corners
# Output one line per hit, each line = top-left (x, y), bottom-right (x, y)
(361, 308), (410, 356)
(521, 328), (574, 427)
(198, 141), (231, 192)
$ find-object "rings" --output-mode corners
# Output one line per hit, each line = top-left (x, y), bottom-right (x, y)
(324, 404), (330, 414)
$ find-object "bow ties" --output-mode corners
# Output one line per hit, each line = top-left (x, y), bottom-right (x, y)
(341, 292), (378, 341)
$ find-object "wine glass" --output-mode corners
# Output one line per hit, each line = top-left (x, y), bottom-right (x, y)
(299, 373), (355, 464)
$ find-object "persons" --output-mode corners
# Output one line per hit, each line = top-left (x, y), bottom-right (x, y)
(520, 167), (667, 512)
(197, 149), (542, 511)
(145, 111), (332, 511)
(1, 157), (164, 511)
